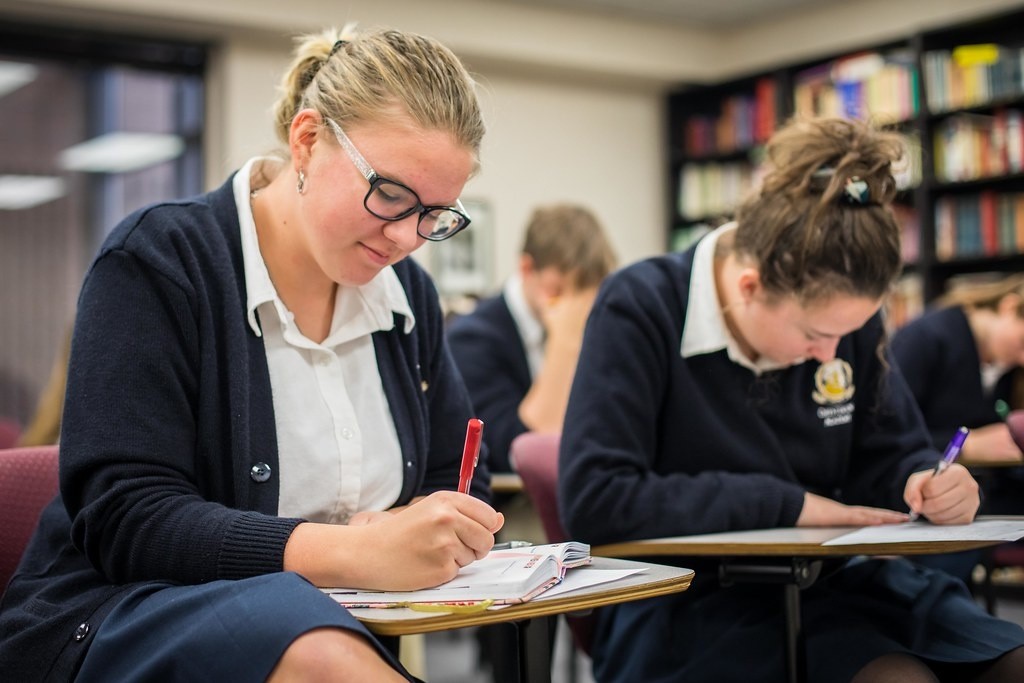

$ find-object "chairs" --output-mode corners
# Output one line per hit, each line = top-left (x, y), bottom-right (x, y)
(507, 430), (596, 683)
(0, 445), (61, 587)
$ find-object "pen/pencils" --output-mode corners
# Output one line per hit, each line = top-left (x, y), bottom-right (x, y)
(490, 541), (533, 549)
(457, 418), (485, 494)
(910, 426), (970, 522)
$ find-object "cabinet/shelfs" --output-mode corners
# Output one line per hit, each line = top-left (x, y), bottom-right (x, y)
(663, 2), (1024, 339)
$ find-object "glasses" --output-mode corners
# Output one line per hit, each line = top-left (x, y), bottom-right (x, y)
(325, 117), (471, 242)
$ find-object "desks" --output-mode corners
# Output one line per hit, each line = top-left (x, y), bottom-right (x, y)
(588, 516), (1024, 683)
(314, 555), (696, 666)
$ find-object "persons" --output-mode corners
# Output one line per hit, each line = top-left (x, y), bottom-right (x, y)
(445, 203), (619, 473)
(556, 118), (1024, 683)
(889, 275), (1024, 520)
(0, 29), (504, 683)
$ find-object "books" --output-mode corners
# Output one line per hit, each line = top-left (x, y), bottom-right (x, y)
(327, 542), (592, 608)
(669, 45), (1024, 329)
